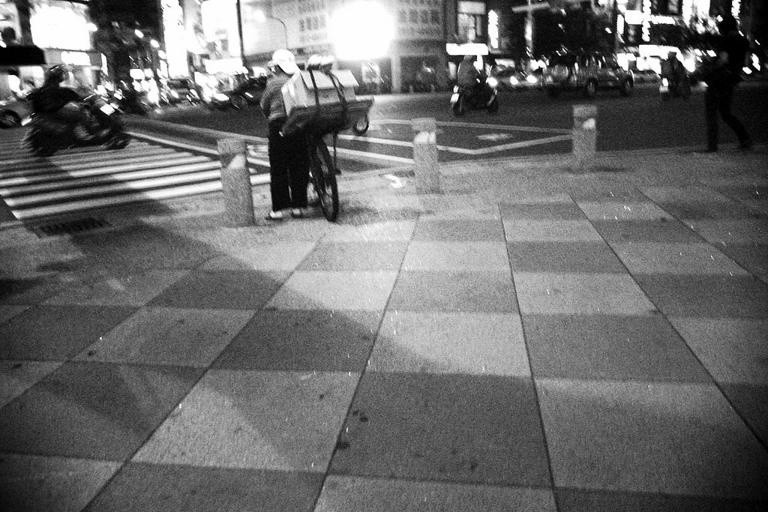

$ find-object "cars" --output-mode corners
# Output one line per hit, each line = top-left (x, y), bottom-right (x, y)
(632, 68), (660, 84)
(0, 74), (271, 126)
(496, 68), (539, 92)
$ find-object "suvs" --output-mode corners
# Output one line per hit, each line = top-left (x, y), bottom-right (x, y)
(541, 50), (635, 100)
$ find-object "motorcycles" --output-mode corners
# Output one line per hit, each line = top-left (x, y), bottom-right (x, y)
(657, 75), (691, 100)
(25, 96), (129, 151)
(451, 83), (499, 116)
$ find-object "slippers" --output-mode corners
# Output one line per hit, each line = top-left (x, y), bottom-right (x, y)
(289, 208), (303, 218)
(265, 213), (284, 221)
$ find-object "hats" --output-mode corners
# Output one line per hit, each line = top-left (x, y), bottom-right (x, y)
(272, 49), (300, 74)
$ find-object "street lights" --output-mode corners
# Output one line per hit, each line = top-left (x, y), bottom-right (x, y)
(251, 10), (290, 57)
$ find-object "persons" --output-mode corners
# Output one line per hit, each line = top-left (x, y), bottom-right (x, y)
(661, 52), (692, 100)
(456, 54), (489, 109)
(690, 47), (755, 155)
(30, 65), (96, 145)
(256, 48), (311, 222)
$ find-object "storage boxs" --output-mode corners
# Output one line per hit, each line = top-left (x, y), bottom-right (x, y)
(279, 68), (359, 117)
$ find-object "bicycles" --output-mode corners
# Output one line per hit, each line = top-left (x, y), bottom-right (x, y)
(304, 133), (341, 222)
